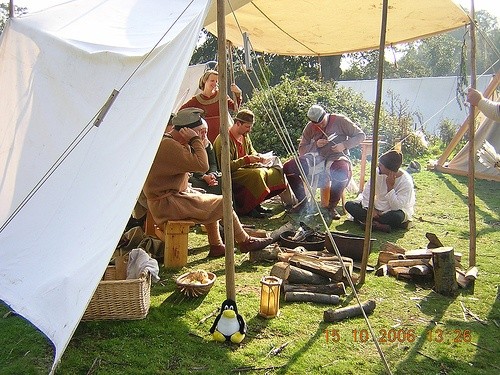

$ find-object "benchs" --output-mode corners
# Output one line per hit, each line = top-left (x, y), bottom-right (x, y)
(145, 211), (195, 270)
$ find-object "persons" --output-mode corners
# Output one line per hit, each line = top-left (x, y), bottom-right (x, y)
(131, 108), (273, 256)
(213, 110), (286, 217)
(284, 105), (366, 218)
(346, 151), (415, 233)
(188, 118), (222, 231)
(179, 69), (242, 147)
(467, 87), (500, 122)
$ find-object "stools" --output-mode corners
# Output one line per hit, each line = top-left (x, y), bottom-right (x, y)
(310, 175), (345, 207)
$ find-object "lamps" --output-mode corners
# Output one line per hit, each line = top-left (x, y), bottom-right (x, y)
(258, 276), (283, 319)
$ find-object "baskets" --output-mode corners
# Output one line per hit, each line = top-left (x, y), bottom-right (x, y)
(176, 271), (216, 297)
(80, 266), (151, 322)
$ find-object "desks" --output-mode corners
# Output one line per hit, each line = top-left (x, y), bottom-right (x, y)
(297, 138), (386, 193)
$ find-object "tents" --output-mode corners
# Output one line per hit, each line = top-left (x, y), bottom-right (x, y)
(0, 0), (476, 375)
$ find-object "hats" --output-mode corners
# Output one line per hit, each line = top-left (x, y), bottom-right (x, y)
(235, 109), (254, 124)
(172, 107), (204, 130)
(307, 105), (323, 123)
(379, 150), (402, 172)
(198, 70), (219, 91)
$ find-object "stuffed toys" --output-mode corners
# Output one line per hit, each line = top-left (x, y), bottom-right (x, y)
(211, 299), (246, 342)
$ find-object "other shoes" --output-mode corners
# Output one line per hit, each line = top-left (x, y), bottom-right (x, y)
(284, 204), (298, 213)
(297, 200), (309, 214)
(354, 218), (366, 230)
(239, 208), (267, 218)
(209, 244), (238, 259)
(328, 208), (341, 220)
(238, 236), (273, 253)
(256, 203), (273, 212)
(375, 223), (391, 233)
